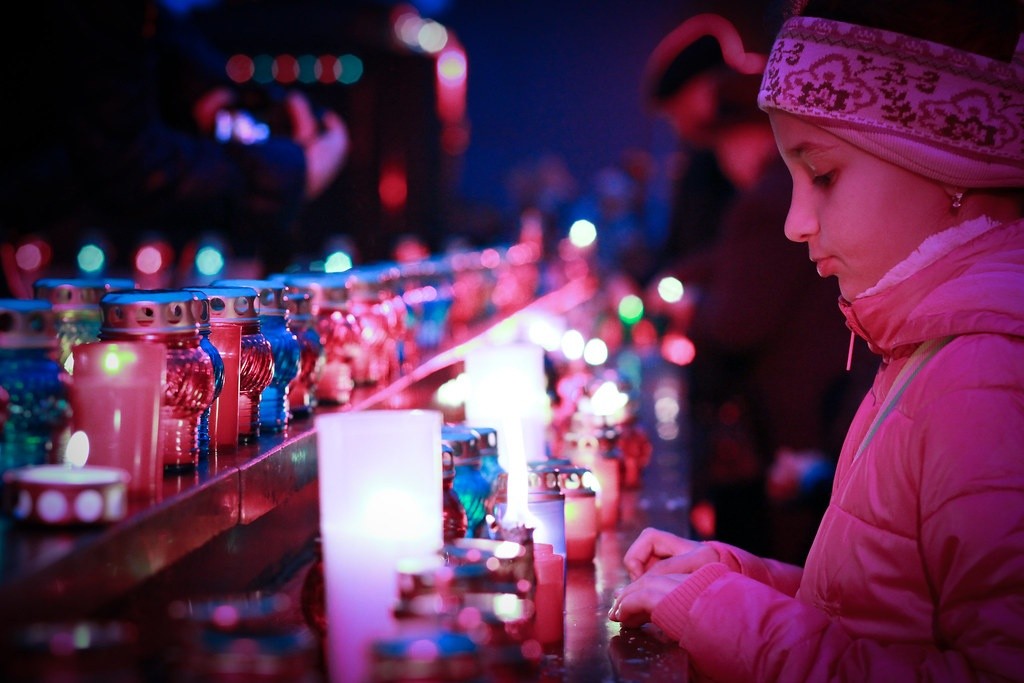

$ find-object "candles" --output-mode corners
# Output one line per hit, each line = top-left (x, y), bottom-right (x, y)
(7, 433), (131, 522)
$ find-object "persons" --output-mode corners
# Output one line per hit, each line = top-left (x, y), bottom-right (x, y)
(604, 0), (1021, 683)
(621, 18), (881, 568)
(0, 0), (466, 288)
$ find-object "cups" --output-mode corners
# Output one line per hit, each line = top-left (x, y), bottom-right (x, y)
(206, 318), (239, 453)
(68, 340), (169, 499)
(527, 490), (568, 593)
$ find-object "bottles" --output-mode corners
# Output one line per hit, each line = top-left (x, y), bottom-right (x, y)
(532, 542), (565, 643)
(0, 258), (608, 506)
(438, 425), (508, 544)
(562, 485), (596, 566)
